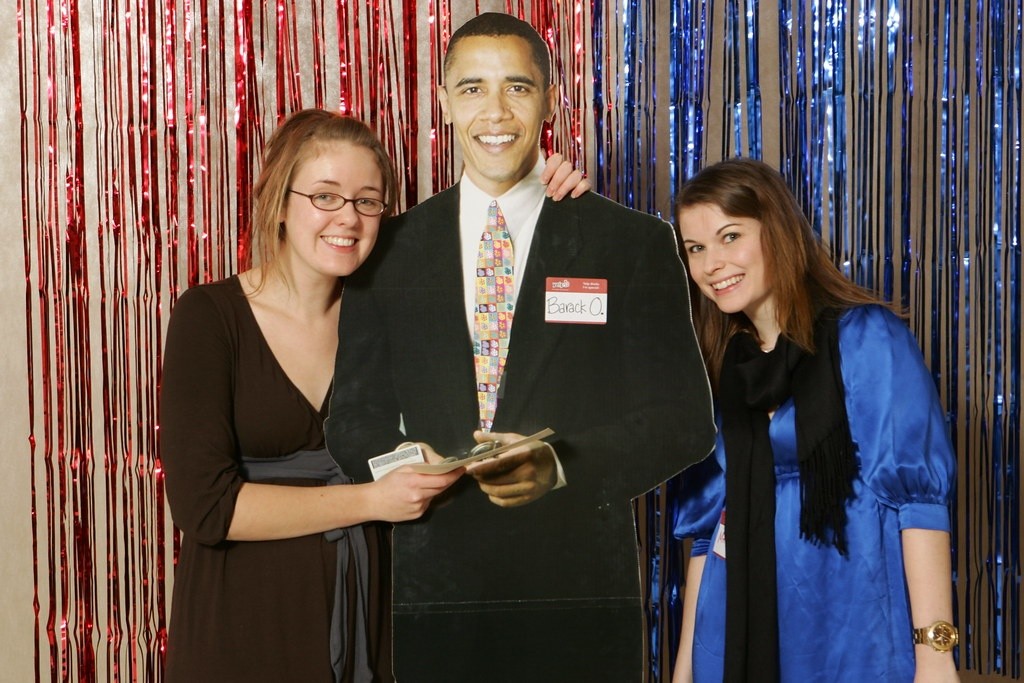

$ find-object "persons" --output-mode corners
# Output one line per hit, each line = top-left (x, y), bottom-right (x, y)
(157, 109), (591, 683)
(672, 158), (963, 683)
(323, 11), (719, 683)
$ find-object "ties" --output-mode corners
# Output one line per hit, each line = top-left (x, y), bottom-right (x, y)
(471, 198), (515, 439)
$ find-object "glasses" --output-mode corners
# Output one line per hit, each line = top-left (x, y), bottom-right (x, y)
(438, 440), (503, 467)
(288, 189), (388, 218)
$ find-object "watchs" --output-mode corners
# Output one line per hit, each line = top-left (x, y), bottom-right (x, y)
(913, 620), (959, 654)
